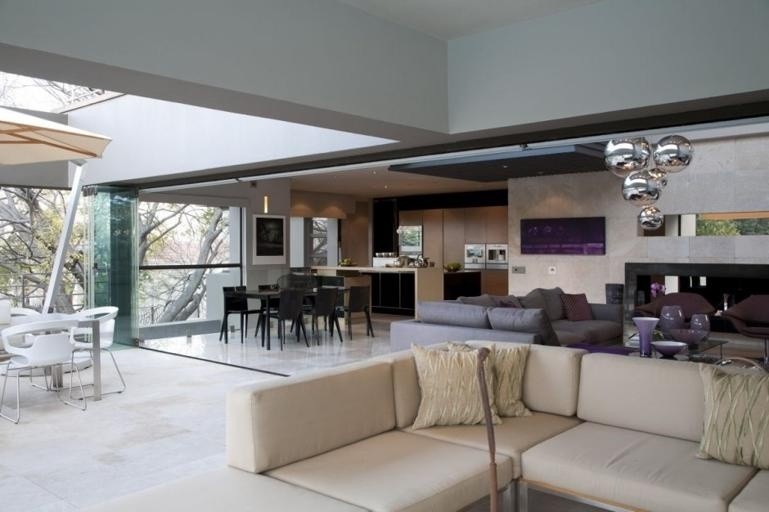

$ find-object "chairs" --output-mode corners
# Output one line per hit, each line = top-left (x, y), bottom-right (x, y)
(635, 292), (715, 327)
(218, 265), (375, 351)
(0, 305), (126, 424)
(721, 295), (769, 339)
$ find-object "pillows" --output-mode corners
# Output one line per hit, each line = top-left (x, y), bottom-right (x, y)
(468, 342), (533, 417)
(411, 340), (503, 431)
(695, 363), (768, 467)
(414, 286), (593, 346)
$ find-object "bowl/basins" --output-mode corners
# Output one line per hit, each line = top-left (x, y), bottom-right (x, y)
(648, 340), (688, 358)
(375, 252), (396, 258)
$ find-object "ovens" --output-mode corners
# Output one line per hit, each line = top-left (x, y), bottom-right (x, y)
(485, 244), (508, 270)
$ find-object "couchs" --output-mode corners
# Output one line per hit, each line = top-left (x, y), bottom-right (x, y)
(86, 337), (769, 512)
(390, 303), (623, 353)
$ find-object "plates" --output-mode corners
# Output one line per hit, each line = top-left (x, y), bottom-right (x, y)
(671, 328), (709, 350)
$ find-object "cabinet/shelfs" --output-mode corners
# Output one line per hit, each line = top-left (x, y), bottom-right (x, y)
(464, 206), (507, 244)
(485, 270), (507, 295)
(423, 208), (464, 269)
(398, 211), (423, 224)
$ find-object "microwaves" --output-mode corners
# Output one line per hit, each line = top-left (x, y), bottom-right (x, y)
(464, 243), (486, 270)
